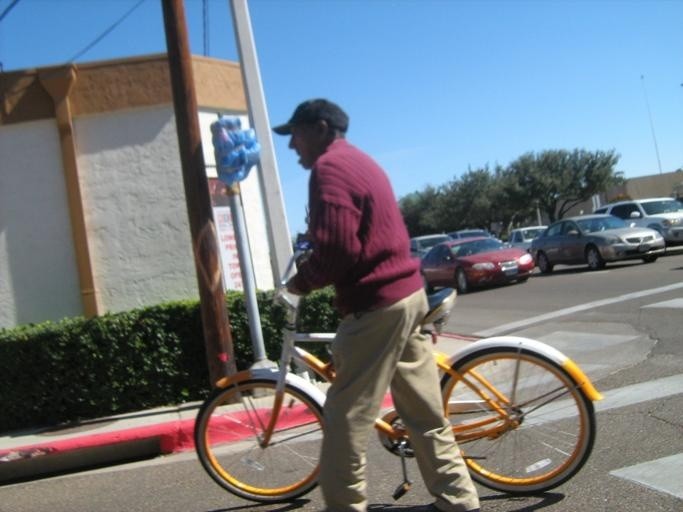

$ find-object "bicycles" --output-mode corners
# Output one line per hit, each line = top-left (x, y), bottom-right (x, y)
(183, 239), (605, 508)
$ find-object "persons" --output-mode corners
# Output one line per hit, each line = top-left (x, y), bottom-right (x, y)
(267, 95), (484, 511)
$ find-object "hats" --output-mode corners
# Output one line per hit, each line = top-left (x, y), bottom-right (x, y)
(270, 98), (349, 133)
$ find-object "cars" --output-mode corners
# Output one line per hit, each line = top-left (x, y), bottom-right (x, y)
(406, 195), (682, 295)
